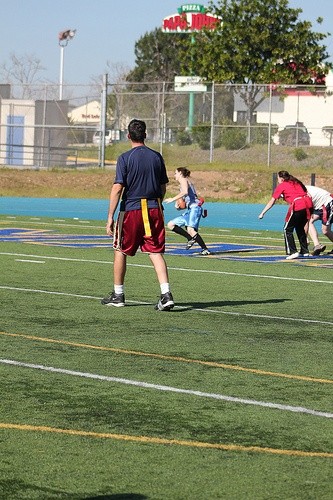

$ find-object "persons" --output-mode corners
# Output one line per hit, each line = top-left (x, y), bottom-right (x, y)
(164, 167), (211, 255)
(101, 119), (175, 311)
(258, 170), (314, 260)
(304, 185), (333, 256)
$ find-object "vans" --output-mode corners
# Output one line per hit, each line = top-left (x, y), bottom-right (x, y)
(92, 130), (120, 145)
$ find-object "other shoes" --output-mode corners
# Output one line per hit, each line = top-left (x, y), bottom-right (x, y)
(311, 244), (326, 256)
(298, 252), (310, 257)
(286, 252), (299, 259)
(328, 249), (333, 257)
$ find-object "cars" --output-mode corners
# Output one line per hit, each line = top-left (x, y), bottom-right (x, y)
(278, 125), (312, 146)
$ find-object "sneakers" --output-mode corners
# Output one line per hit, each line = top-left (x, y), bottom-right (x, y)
(185, 238), (196, 250)
(153, 291), (175, 311)
(101, 291), (125, 307)
(198, 249), (211, 255)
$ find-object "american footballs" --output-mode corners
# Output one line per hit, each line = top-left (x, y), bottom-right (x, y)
(177, 197), (187, 209)
